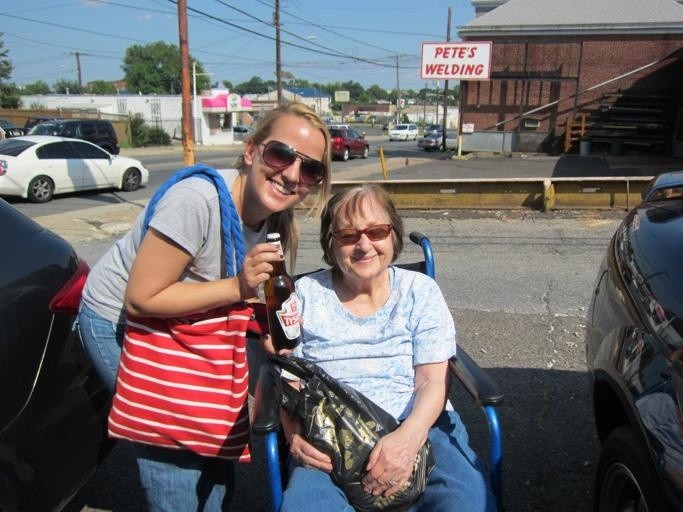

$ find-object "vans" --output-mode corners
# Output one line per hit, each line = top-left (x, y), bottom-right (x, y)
(386, 122), (419, 142)
(25, 116), (121, 157)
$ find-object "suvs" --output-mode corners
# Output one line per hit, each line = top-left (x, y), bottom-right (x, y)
(423, 125), (443, 138)
(0, 194), (127, 511)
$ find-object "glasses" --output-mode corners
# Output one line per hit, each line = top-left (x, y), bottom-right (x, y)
(331, 224), (393, 245)
(261, 140), (325, 186)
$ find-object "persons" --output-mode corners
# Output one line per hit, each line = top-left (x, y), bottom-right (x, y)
(279, 184), (498, 512)
(70, 102), (332, 512)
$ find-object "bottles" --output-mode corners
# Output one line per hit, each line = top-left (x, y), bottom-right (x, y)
(262, 231), (301, 353)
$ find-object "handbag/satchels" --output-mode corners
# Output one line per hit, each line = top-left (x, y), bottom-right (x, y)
(107, 301), (254, 463)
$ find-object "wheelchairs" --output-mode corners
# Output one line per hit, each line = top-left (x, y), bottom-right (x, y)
(252, 230), (512, 512)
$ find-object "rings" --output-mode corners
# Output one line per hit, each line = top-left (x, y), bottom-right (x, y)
(387, 480), (396, 488)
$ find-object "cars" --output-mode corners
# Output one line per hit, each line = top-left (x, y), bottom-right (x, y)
(326, 126), (371, 161)
(0, 135), (153, 206)
(418, 132), (458, 152)
(233, 125), (255, 140)
(581, 167), (681, 512)
(0, 115), (65, 141)
